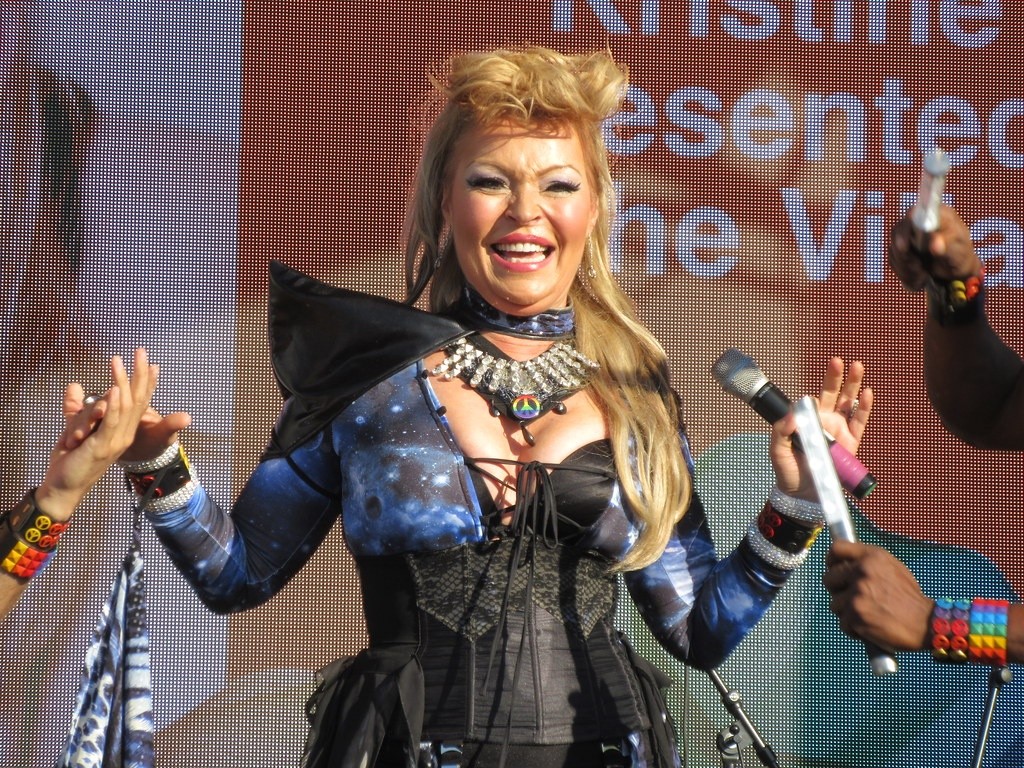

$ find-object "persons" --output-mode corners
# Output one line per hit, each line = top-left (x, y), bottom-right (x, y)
(0, 347), (159, 621)
(69, 45), (875, 768)
(823, 545), (1024, 668)
(886, 202), (1023, 452)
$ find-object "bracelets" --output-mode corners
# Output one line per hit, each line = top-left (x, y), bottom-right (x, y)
(0, 487), (72, 582)
(749, 490), (826, 570)
(928, 265), (985, 326)
(931, 596), (1007, 667)
(118, 442), (197, 516)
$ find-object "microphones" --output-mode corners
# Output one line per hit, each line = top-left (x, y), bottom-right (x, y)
(710, 347), (877, 500)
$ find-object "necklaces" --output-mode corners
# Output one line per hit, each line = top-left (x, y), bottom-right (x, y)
(461, 285), (576, 341)
(435, 336), (599, 447)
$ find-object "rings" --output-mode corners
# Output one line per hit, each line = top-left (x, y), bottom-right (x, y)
(837, 407), (852, 417)
(83, 391), (101, 405)
